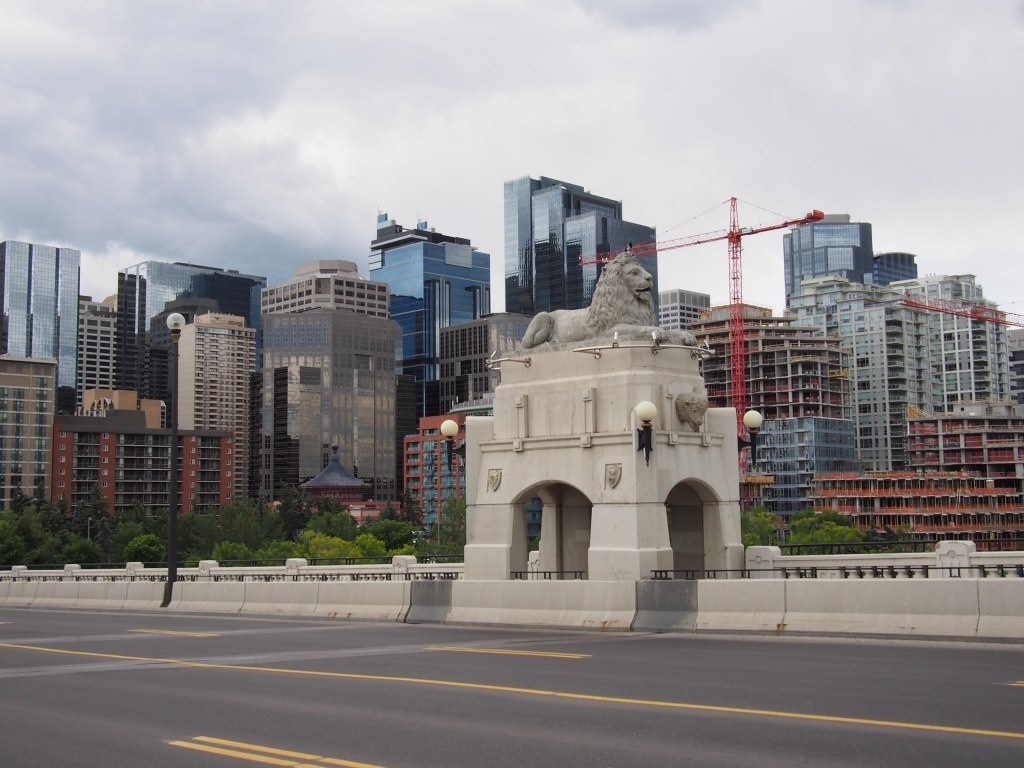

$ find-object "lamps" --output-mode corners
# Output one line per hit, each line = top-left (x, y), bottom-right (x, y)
(703, 340), (710, 352)
(652, 331), (659, 347)
(441, 420), (466, 472)
(612, 332), (620, 348)
(737, 410), (762, 466)
(491, 350), (499, 360)
(636, 401), (657, 466)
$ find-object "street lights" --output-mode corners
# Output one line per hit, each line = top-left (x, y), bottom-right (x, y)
(88, 516), (92, 540)
(167, 313), (185, 582)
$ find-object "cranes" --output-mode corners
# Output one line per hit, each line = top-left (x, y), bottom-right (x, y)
(900, 291), (1024, 329)
(577, 196), (824, 507)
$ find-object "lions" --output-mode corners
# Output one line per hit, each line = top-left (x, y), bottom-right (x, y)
(521, 252), (697, 348)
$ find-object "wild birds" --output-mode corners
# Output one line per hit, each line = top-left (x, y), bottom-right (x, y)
(625, 243), (632, 251)
